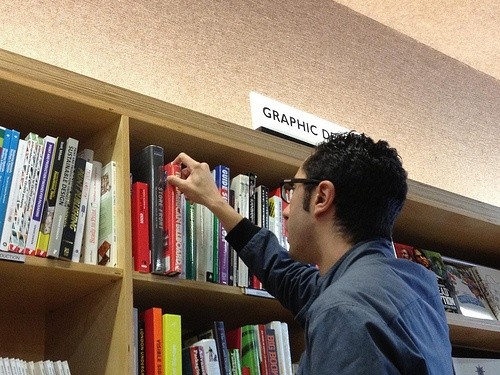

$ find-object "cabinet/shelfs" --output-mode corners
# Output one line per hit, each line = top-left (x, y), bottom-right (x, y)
(0, 48), (500, 374)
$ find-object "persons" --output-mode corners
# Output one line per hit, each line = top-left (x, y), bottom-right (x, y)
(165, 130), (457, 375)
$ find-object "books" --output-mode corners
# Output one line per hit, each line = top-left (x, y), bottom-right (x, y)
(130, 143), (293, 289)
(79, 160), (102, 266)
(0, 125), (79, 259)
(59, 159), (94, 262)
(76, 148), (95, 161)
(97, 161), (117, 266)
(452, 356), (500, 375)
(133, 306), (302, 375)
(0, 356), (72, 375)
(390, 242), (500, 321)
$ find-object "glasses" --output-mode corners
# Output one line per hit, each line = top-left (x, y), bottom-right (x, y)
(280, 179), (322, 204)
